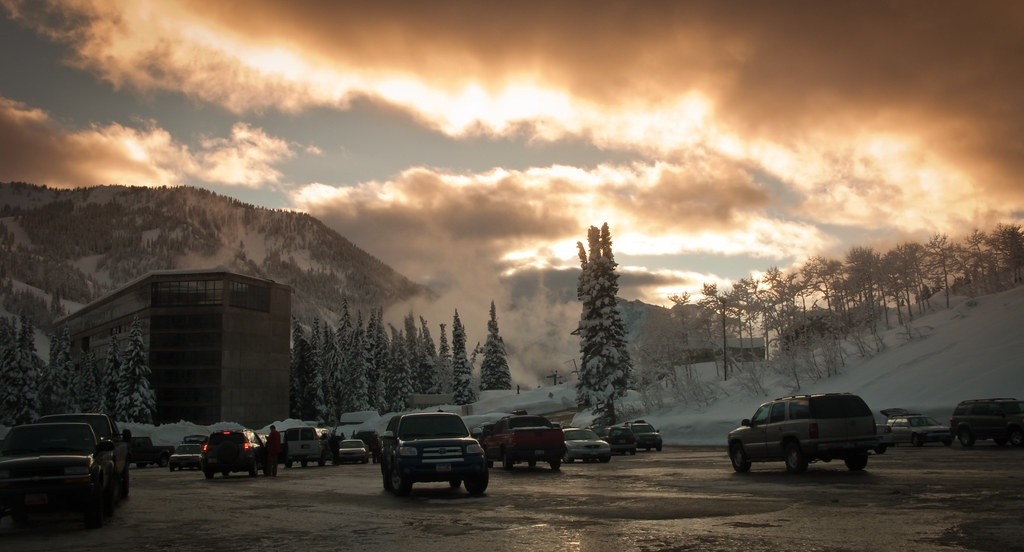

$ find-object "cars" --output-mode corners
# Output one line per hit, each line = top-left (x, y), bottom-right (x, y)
(0, 422), (116, 530)
(881, 406), (954, 447)
(873, 423), (897, 455)
(338, 439), (370, 464)
(168, 434), (209, 472)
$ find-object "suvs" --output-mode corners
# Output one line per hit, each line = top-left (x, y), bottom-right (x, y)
(378, 411), (490, 498)
(949, 396), (1024, 448)
(283, 426), (333, 468)
(469, 409), (664, 472)
(34, 412), (133, 504)
(199, 428), (265, 479)
(727, 391), (885, 477)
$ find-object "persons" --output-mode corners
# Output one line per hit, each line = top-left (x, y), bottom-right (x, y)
(327, 432), (340, 466)
(263, 425), (281, 477)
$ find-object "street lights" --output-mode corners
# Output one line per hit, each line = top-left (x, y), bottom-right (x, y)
(720, 298), (728, 382)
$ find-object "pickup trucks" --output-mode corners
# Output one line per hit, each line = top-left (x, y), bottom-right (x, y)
(127, 436), (176, 468)
